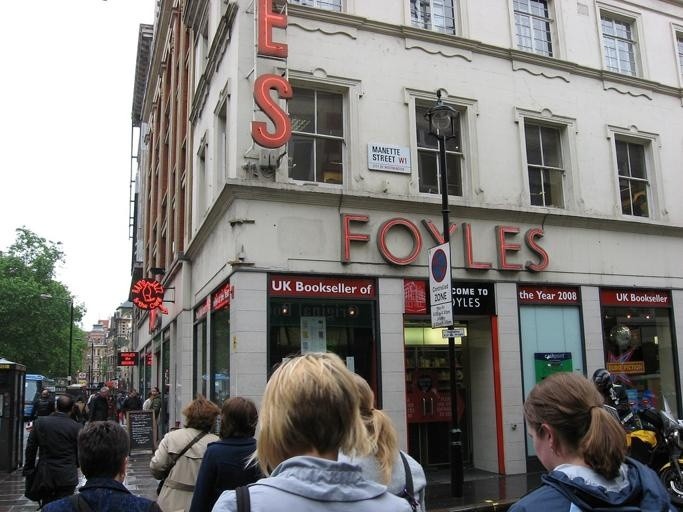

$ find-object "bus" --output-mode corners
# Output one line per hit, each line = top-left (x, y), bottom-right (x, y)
(24, 373), (47, 418)
(48, 378), (55, 392)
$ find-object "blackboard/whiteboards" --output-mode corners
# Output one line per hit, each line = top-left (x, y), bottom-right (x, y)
(126, 409), (157, 450)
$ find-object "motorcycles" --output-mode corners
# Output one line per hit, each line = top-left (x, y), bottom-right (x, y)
(623, 407), (682, 506)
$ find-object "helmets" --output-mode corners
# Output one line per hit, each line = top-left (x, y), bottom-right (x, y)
(594, 369), (613, 389)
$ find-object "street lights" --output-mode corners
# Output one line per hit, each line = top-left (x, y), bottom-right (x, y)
(421, 89), (464, 502)
(40, 293), (74, 386)
(75, 337), (94, 382)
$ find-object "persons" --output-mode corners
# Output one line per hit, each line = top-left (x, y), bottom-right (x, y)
(149, 400), (221, 512)
(588, 367), (635, 434)
(496, 371), (677, 511)
(189, 397), (266, 512)
(18, 379), (167, 512)
(337, 373), (426, 512)
(212, 354), (414, 512)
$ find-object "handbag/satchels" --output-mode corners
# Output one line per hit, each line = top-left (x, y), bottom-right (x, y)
(156, 477), (164, 495)
(25, 455), (52, 501)
